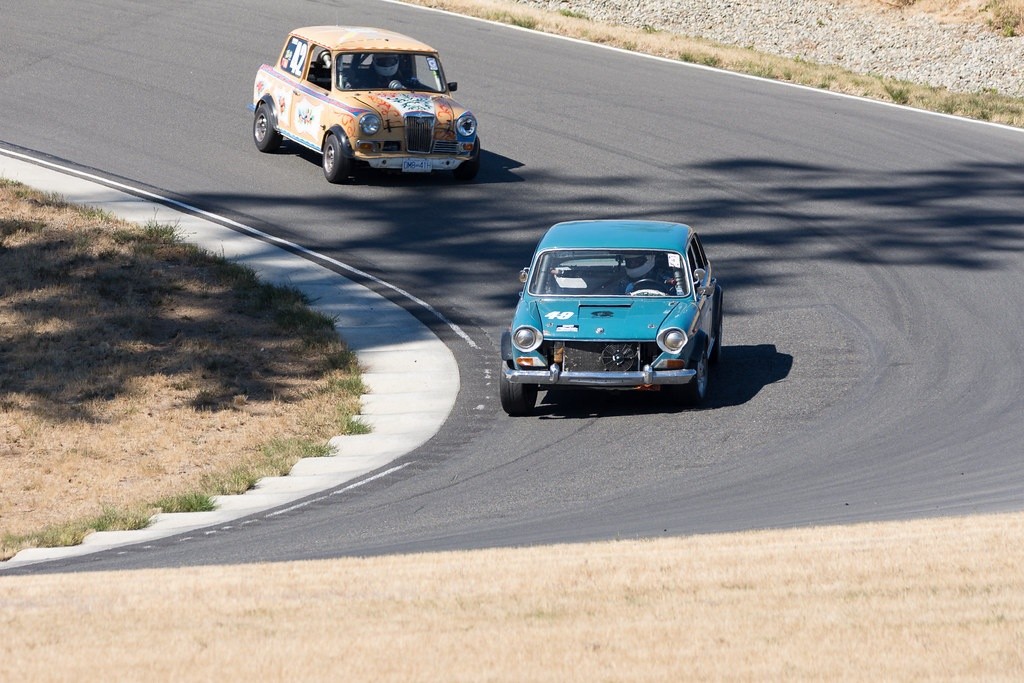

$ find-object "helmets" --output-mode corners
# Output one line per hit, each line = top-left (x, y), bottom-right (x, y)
(372, 53), (399, 76)
(623, 253), (656, 278)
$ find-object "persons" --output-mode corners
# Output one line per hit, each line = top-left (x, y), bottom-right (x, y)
(618, 251), (683, 298)
(363, 53), (410, 89)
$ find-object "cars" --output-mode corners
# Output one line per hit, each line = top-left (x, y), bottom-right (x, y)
(498, 218), (724, 416)
(252, 25), (480, 185)
